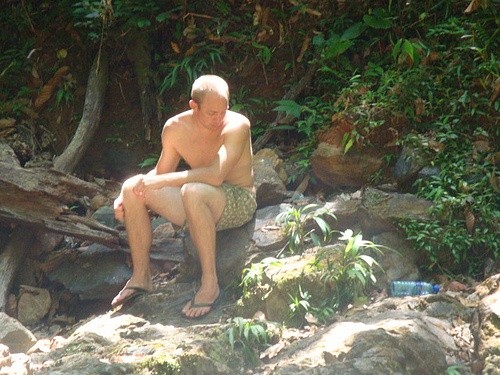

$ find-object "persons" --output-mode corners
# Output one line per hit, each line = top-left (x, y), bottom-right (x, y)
(111, 74), (258, 321)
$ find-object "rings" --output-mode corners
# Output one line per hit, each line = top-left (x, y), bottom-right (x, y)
(139, 191), (143, 196)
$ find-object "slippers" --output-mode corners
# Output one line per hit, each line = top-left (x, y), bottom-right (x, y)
(180, 288), (224, 321)
(111, 286), (148, 309)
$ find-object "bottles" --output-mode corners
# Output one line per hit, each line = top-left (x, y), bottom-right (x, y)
(391, 281), (439, 296)
